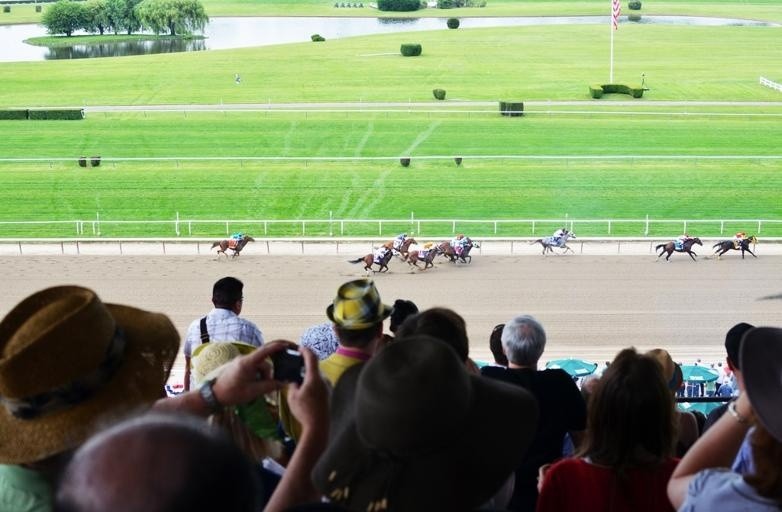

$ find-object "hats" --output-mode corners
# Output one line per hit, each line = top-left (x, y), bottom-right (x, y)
(188, 342), (276, 387)
(644, 348), (683, 390)
(308, 332), (531, 512)
(325, 277), (395, 332)
(740, 326), (782, 442)
(2, 282), (182, 470)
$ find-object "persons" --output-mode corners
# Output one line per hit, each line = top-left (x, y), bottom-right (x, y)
(422, 243), (433, 260)
(553, 228), (567, 244)
(374, 244), (389, 262)
(231, 234), (242, 247)
(185, 277), (782, 512)
(56, 406), (263, 510)
(234, 74), (241, 85)
(1, 285), (334, 510)
(733, 232), (746, 249)
(452, 234), (465, 255)
(678, 235), (693, 247)
(395, 234), (407, 251)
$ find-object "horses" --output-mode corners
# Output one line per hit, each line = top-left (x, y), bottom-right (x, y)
(712, 235), (759, 260)
(400, 245), (445, 273)
(655, 236), (703, 263)
(528, 231), (577, 255)
(210, 235), (255, 259)
(374, 238), (418, 261)
(439, 237), (480, 264)
(347, 249), (400, 277)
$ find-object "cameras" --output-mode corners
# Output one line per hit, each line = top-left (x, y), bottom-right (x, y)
(269, 348), (304, 385)
